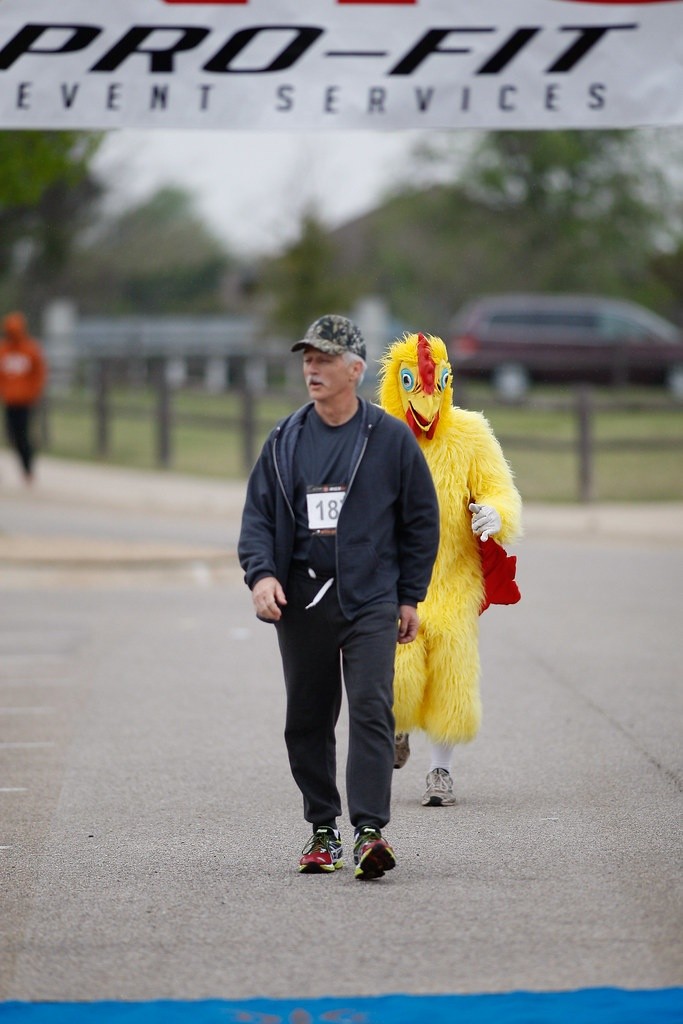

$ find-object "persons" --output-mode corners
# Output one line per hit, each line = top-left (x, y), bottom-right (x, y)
(0, 313), (45, 480)
(237, 314), (440, 878)
(379, 332), (522, 805)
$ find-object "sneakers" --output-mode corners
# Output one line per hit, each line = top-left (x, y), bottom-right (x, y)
(353, 826), (396, 880)
(394, 733), (410, 769)
(299, 826), (346, 873)
(421, 768), (456, 806)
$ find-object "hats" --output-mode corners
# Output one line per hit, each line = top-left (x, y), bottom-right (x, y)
(290, 314), (366, 361)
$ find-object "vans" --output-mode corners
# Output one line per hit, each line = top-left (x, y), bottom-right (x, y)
(447, 297), (683, 408)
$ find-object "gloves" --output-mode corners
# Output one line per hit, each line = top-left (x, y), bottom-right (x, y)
(469, 503), (501, 541)
